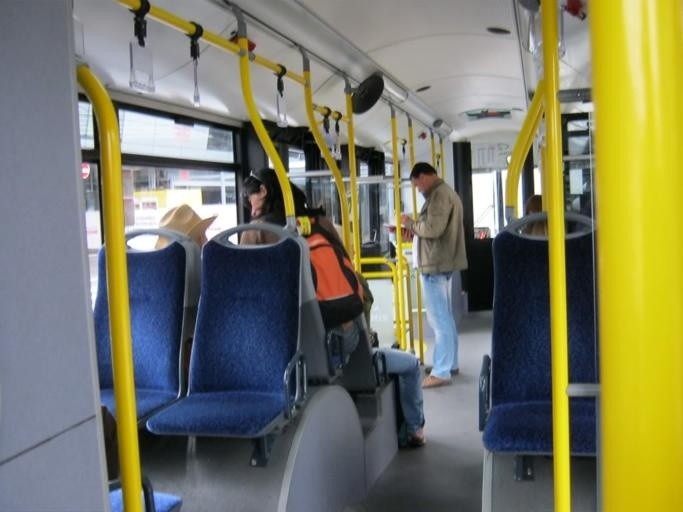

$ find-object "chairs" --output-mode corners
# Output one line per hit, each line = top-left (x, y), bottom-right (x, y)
(478, 212), (599, 483)
(93, 221), (396, 512)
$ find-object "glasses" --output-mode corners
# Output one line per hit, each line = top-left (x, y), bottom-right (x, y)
(249, 166), (263, 183)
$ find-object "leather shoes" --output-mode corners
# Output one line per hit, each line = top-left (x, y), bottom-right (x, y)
(420, 364), (460, 389)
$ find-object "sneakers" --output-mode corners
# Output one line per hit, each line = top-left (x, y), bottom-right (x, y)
(405, 427), (426, 447)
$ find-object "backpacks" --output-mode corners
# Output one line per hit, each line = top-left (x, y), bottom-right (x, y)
(257, 205), (365, 329)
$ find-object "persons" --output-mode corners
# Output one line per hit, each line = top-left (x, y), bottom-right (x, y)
(389, 162), (469, 389)
(239, 168), (425, 448)
(522, 195), (545, 235)
